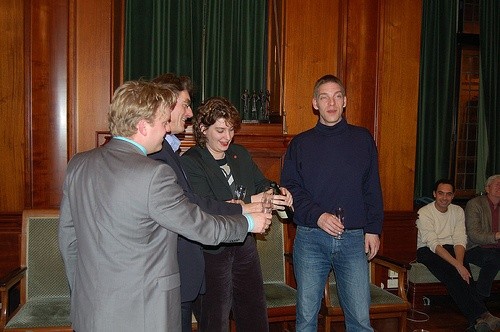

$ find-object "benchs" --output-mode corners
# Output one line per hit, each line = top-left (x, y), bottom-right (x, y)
(407, 263), (500, 314)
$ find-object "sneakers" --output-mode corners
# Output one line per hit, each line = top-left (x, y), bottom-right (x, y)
(475, 312), (500, 332)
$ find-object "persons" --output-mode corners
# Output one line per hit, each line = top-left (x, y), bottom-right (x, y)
(280, 74), (384, 332)
(146, 73), (274, 332)
(240, 89), (272, 124)
(464, 175), (500, 309)
(179, 96), (294, 332)
(57, 79), (272, 332)
(414, 178), (500, 332)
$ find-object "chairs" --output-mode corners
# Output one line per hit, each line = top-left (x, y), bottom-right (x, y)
(0, 210), (411, 332)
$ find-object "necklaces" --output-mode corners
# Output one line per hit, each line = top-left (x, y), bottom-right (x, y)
(213, 153), (225, 158)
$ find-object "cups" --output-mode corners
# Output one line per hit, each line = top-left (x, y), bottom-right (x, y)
(234, 185), (247, 201)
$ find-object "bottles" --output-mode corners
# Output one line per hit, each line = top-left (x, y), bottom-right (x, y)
(271, 183), (295, 226)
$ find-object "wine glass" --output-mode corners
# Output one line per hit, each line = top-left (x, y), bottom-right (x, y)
(334, 208), (346, 240)
(257, 186), (274, 241)
